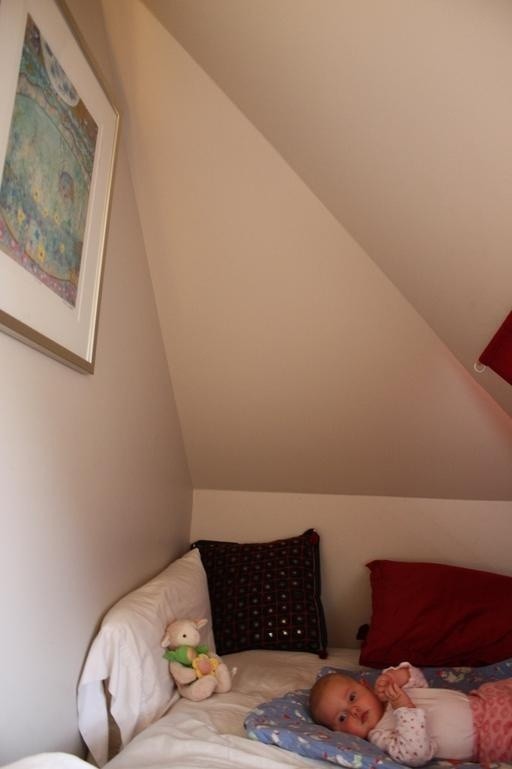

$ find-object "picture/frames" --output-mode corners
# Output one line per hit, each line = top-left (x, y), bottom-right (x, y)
(0, 0), (123, 376)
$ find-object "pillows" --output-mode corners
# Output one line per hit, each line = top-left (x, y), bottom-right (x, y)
(357, 559), (511, 671)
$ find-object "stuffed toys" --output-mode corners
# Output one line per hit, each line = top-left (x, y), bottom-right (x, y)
(160, 614), (236, 702)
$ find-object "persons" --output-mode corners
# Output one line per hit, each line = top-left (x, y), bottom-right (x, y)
(307, 661), (512, 768)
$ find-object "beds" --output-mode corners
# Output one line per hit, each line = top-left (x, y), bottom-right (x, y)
(76, 488), (511, 769)
